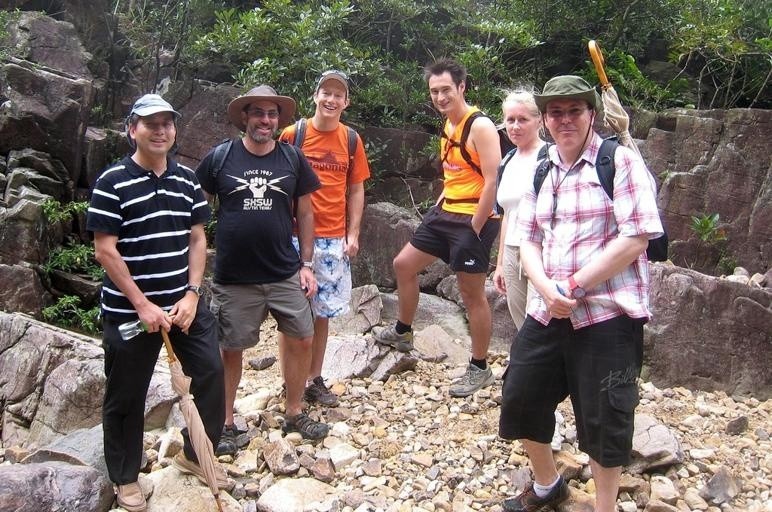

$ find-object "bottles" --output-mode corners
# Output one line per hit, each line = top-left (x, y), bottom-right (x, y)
(118, 310), (171, 342)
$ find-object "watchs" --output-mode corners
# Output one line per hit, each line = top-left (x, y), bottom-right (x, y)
(301, 261), (314, 270)
(185, 285), (205, 297)
(568, 275), (587, 299)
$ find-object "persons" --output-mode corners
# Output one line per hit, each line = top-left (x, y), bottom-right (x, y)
(277, 70), (370, 409)
(86, 94), (228, 512)
(499, 75), (665, 512)
(193, 84), (329, 457)
(373, 59), (502, 398)
(494, 86), (565, 454)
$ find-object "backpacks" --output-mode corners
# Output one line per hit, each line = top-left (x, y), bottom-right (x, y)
(294, 117), (357, 178)
(534, 140), (668, 262)
(438, 112), (515, 215)
(497, 143), (556, 185)
(211, 137), (301, 204)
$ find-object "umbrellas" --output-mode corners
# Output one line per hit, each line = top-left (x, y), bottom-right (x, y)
(588, 39), (642, 159)
(161, 315), (225, 512)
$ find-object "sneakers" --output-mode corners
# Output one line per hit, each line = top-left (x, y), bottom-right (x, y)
(449, 362), (494, 398)
(502, 475), (571, 512)
(213, 422), (239, 455)
(116, 476), (149, 511)
(305, 378), (340, 407)
(282, 415), (328, 438)
(371, 325), (413, 351)
(173, 451), (228, 489)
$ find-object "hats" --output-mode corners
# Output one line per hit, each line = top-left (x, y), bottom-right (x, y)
(533, 76), (603, 115)
(228, 85), (295, 133)
(125, 93), (180, 123)
(316, 70), (350, 99)
